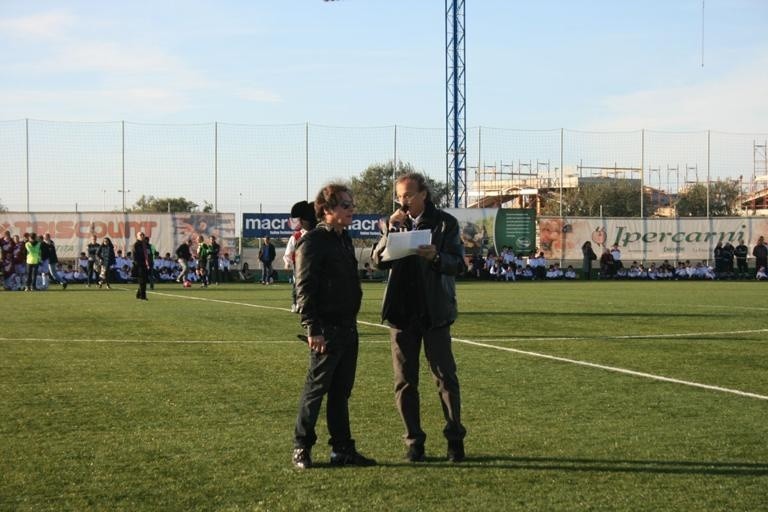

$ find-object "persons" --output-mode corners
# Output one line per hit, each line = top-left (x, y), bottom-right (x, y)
(459, 235), (764, 280)
(291, 182), (377, 469)
(369, 173), (468, 463)
(282, 225), (311, 313)
(1, 230), (279, 300)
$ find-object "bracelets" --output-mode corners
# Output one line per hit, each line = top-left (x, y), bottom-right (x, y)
(434, 252), (440, 263)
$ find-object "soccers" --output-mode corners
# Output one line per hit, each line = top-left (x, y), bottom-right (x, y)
(184, 281), (191, 287)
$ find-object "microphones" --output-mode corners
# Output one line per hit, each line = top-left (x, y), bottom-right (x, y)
(392, 205), (409, 231)
(298, 333), (342, 356)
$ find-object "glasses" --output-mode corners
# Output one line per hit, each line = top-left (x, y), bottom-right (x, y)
(395, 192), (419, 203)
(338, 199), (355, 210)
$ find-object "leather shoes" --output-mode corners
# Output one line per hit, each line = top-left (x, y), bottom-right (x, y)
(407, 445), (425, 462)
(446, 439), (466, 462)
(330, 450), (377, 468)
(291, 448), (313, 469)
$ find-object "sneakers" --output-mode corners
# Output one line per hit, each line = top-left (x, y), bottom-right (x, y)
(265, 281), (269, 285)
(262, 280), (265, 284)
